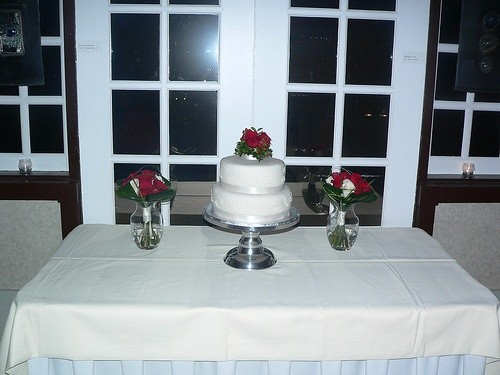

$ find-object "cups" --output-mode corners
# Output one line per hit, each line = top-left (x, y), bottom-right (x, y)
(462, 162), (475, 178)
(18, 158), (32, 174)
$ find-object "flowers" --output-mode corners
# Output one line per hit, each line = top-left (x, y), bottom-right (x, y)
(322, 166), (377, 250)
(113, 170), (177, 247)
(233, 126), (274, 162)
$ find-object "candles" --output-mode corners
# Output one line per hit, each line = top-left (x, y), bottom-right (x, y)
(20, 161), (31, 170)
(464, 164), (473, 175)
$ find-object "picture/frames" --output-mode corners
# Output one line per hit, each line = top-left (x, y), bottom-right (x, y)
(0, 0), (45, 87)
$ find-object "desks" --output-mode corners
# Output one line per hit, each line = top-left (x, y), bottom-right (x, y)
(0, 222), (500, 375)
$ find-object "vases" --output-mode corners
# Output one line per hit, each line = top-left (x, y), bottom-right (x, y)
(128, 200), (164, 251)
(325, 202), (360, 252)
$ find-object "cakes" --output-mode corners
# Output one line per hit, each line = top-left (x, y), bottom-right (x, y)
(211, 155), (292, 221)
